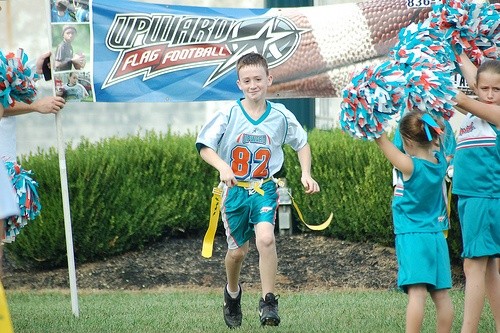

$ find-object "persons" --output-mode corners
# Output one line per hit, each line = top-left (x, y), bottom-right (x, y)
(52, 0), (88, 22)
(373, 47), (500, 333)
(0, 93), (66, 254)
(194, 52), (321, 329)
(56, 72), (90, 100)
(54, 25), (86, 70)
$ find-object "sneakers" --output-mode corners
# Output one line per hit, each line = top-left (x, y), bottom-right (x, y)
(258, 293), (280, 326)
(223, 283), (242, 329)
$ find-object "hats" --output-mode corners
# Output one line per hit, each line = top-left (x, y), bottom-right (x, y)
(62, 25), (78, 34)
